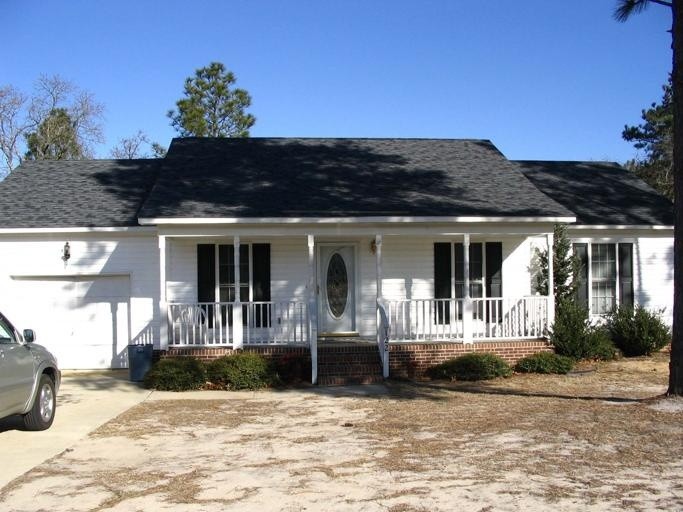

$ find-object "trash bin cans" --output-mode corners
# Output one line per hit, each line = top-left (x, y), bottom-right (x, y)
(128, 344), (153, 381)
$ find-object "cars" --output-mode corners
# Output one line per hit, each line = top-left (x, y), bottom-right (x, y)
(0, 311), (62, 431)
